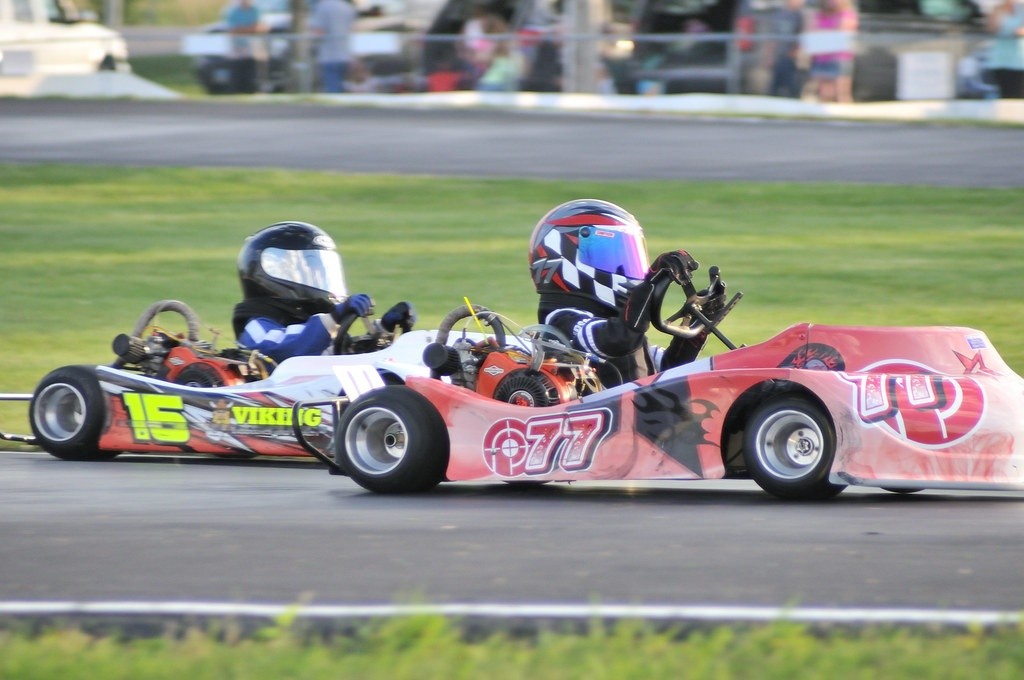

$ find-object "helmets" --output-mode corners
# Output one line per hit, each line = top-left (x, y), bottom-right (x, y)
(529, 198), (650, 314)
(237, 221), (349, 307)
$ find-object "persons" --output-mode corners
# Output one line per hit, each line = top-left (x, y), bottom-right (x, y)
(767, 0), (857, 102)
(223, 1), (269, 94)
(987, 1), (1022, 99)
(231, 222), (416, 365)
(527, 198), (726, 389)
(309, 0), (354, 90)
(458, 10), (630, 92)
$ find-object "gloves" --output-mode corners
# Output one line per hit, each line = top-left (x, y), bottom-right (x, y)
(620, 250), (699, 333)
(664, 282), (725, 367)
(330, 293), (375, 318)
(381, 301), (414, 334)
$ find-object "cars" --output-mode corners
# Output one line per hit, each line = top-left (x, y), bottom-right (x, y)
(0, 0), (133, 82)
